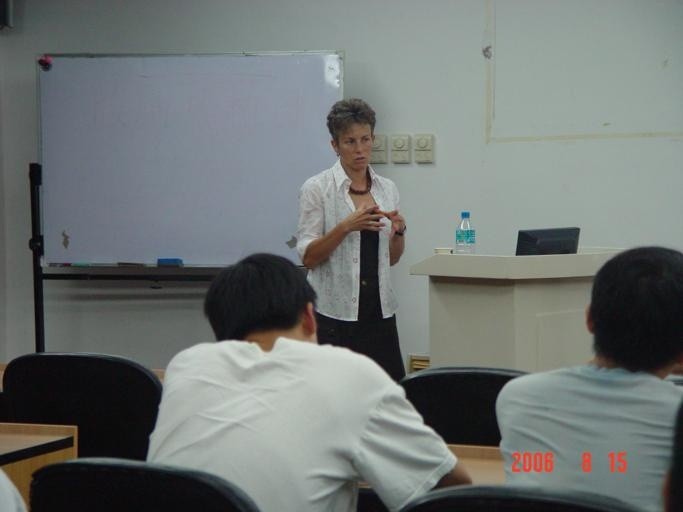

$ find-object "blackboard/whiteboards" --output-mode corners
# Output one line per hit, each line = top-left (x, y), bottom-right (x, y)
(36, 49), (346, 280)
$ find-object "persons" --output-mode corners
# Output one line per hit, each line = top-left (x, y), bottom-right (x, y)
(294, 97), (408, 382)
(144, 252), (474, 511)
(491, 245), (682, 512)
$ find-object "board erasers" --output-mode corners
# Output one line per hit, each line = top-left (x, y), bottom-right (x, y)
(157, 258), (184, 267)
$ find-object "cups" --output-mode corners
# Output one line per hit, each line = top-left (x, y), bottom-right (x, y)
(433, 248), (453, 254)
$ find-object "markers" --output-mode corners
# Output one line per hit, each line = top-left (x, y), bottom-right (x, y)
(63, 263), (89, 267)
(118, 263), (144, 267)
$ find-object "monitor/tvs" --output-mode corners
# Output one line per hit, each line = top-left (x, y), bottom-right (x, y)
(516, 227), (580, 255)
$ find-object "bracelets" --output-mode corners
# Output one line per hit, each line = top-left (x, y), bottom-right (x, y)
(394, 225), (407, 236)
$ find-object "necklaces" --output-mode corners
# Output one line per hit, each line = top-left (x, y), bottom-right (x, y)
(348, 171), (372, 195)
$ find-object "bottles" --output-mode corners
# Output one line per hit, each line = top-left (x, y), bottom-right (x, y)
(455, 212), (476, 253)
